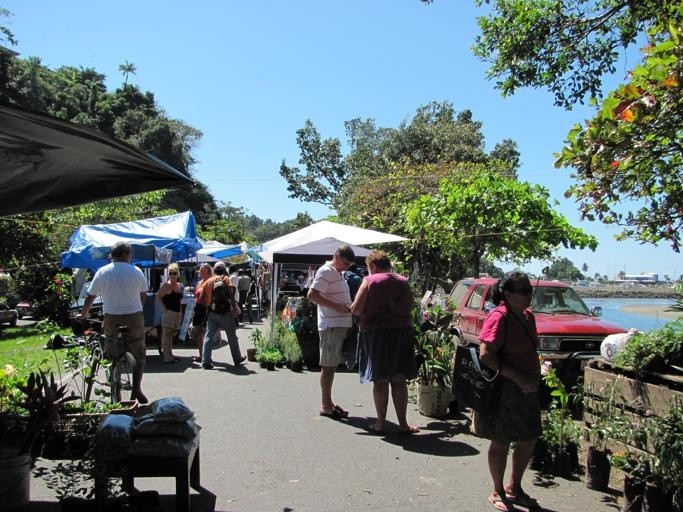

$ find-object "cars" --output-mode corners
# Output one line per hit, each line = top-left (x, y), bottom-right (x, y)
(0, 302), (19, 326)
(14, 301), (39, 320)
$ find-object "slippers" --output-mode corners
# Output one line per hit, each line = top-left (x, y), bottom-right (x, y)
(399, 427), (419, 433)
(487, 497), (513, 512)
(505, 492), (538, 508)
(368, 424), (384, 435)
(319, 410), (341, 421)
(334, 405), (347, 417)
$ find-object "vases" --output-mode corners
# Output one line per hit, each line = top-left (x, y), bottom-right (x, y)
(549, 444), (577, 474)
(415, 378), (451, 419)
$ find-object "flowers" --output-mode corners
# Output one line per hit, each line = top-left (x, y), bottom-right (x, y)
(415, 295), (461, 382)
(539, 354), (571, 451)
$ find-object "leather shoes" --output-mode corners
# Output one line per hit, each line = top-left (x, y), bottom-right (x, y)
(131, 388), (147, 404)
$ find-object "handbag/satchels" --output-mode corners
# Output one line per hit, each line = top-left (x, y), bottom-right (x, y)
(452, 343), (503, 415)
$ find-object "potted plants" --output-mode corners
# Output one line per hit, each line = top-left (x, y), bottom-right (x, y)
(574, 375), (682, 512)
(0, 310), (139, 458)
(247, 313), (302, 372)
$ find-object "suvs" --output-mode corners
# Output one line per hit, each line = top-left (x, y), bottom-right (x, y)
(439, 276), (635, 407)
(68, 281), (105, 333)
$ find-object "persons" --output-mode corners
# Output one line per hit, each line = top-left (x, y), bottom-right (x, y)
(305, 244), (356, 420)
(470, 270), (552, 511)
(155, 262), (365, 370)
(348, 249), (418, 433)
(72, 241), (149, 404)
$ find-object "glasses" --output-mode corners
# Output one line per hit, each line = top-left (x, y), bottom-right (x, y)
(510, 286), (532, 295)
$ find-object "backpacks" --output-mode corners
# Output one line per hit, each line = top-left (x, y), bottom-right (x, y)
(209, 274), (230, 314)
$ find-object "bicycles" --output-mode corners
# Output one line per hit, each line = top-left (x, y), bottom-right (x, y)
(79, 316), (139, 405)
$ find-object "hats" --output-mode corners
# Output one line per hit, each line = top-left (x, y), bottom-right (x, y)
(213, 262), (225, 270)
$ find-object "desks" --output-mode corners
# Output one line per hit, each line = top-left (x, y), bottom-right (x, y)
(92, 430), (201, 512)
